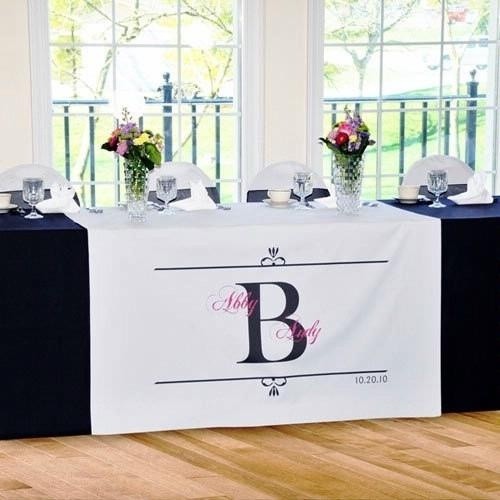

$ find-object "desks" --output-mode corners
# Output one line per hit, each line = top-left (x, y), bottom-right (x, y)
(1, 196), (499, 438)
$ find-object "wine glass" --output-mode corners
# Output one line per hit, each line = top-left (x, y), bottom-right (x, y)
(292, 173), (315, 211)
(155, 176), (178, 217)
(426, 170), (448, 209)
(22, 178), (44, 220)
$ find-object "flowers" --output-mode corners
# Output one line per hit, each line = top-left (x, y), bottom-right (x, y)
(99, 105), (164, 200)
(319, 103), (375, 194)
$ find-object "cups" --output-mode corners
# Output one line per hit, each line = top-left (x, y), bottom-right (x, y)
(266, 188), (291, 203)
(393, 183), (421, 198)
(0, 194), (11, 208)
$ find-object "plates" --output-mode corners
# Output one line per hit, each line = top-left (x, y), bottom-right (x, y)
(0, 204), (18, 214)
(262, 199), (297, 208)
(392, 197), (423, 204)
